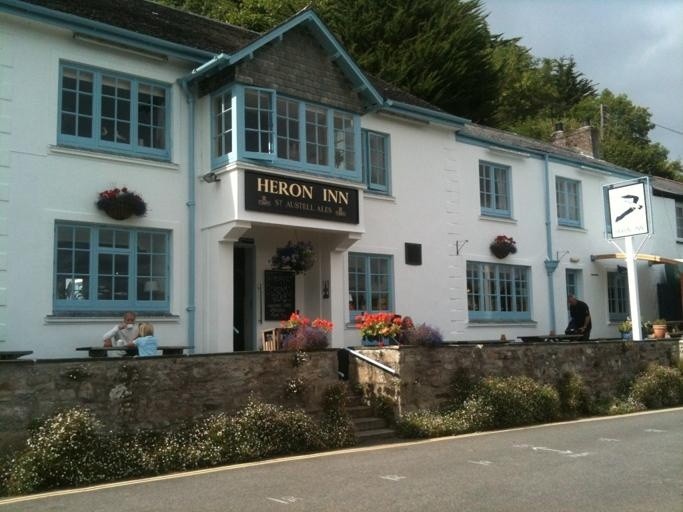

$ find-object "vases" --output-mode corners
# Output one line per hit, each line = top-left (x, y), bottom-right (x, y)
(361, 336), (390, 346)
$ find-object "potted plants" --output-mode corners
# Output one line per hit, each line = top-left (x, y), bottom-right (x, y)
(653, 319), (668, 339)
(618, 320), (633, 338)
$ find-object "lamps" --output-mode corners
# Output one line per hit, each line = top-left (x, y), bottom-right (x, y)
(204, 172), (221, 183)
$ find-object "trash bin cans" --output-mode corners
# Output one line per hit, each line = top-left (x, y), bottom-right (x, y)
(262, 328), (296, 352)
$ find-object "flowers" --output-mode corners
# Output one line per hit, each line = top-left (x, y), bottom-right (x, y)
(354, 311), (401, 340)
(490, 235), (517, 254)
(279, 312), (332, 334)
(98, 187), (148, 216)
(277, 240), (314, 270)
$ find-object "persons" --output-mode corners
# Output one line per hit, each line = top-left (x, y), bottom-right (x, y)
(128, 322), (158, 357)
(102, 310), (138, 347)
(565, 293), (592, 341)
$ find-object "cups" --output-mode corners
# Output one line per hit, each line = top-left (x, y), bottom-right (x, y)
(117, 339), (124, 347)
(127, 323), (134, 330)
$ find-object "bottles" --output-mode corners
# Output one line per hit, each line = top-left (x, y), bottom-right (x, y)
(622, 312), (632, 341)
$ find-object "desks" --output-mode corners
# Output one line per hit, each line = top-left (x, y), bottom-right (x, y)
(516, 333), (584, 342)
